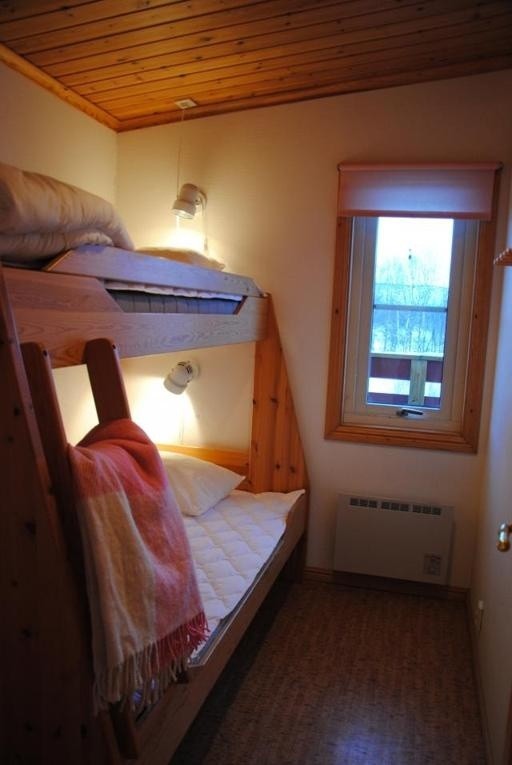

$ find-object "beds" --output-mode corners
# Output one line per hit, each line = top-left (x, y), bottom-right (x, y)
(2, 160), (310, 765)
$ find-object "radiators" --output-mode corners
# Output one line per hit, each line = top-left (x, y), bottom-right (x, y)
(333, 491), (455, 586)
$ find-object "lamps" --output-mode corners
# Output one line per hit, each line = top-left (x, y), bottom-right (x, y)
(163, 359), (199, 395)
(170, 182), (206, 219)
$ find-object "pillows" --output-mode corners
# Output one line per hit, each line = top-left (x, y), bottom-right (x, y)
(159, 451), (246, 517)
(135, 249), (225, 271)
